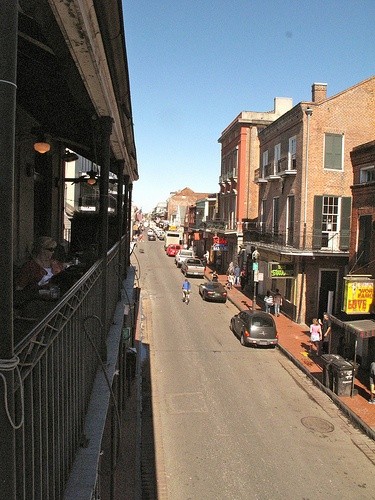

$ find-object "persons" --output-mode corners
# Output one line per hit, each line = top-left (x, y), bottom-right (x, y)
(225, 272), (234, 290)
(309, 318), (322, 356)
(368, 355), (375, 404)
(234, 265), (241, 286)
(264, 290), (273, 314)
(319, 312), (332, 355)
(15, 234), (62, 291)
(182, 279), (191, 302)
(274, 289), (282, 317)
(212, 271), (218, 283)
(337, 337), (346, 357)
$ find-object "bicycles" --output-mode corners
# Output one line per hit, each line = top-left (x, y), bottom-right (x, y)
(182, 288), (190, 305)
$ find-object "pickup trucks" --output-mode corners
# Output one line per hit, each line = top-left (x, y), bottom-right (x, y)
(181, 258), (206, 278)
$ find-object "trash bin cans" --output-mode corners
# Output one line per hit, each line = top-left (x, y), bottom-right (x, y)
(321, 353), (360, 396)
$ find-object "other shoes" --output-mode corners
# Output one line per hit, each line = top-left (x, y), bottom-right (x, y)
(368, 399), (375, 404)
(369, 398), (375, 402)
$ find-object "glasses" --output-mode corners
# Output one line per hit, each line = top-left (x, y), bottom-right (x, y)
(45, 247), (54, 252)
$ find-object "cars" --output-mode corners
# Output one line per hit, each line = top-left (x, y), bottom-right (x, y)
(147, 230), (153, 235)
(229, 310), (279, 348)
(149, 221), (165, 240)
(175, 250), (195, 268)
(167, 244), (182, 257)
(199, 281), (227, 303)
(148, 235), (156, 241)
